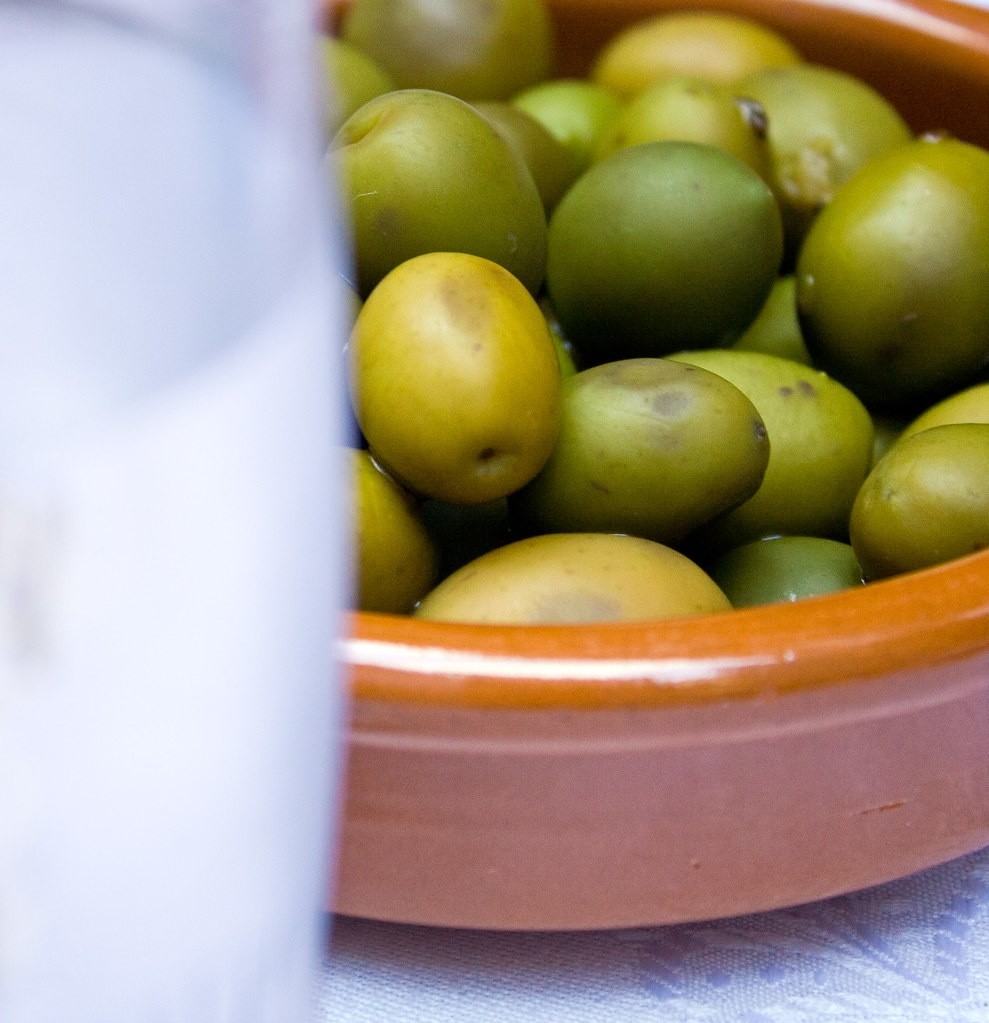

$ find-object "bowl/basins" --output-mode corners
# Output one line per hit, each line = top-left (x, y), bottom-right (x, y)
(301, 0), (989, 931)
